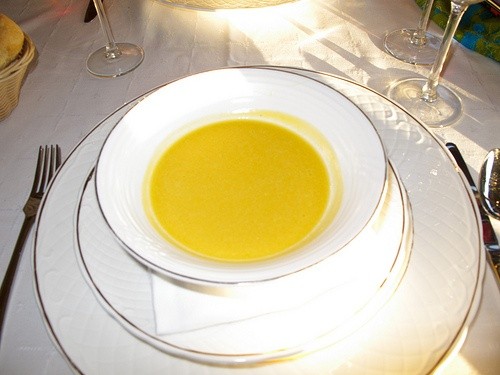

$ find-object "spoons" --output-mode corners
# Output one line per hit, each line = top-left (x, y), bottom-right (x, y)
(479, 149), (500, 219)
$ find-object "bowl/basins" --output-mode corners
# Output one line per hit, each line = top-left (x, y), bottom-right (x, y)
(95, 66), (388, 287)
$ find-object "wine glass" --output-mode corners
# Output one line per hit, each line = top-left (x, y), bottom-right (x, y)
(86, 0), (144, 78)
(384, 0), (451, 65)
(387, 0), (486, 127)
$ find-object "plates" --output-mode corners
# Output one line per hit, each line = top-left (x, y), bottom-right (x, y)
(31, 64), (485, 375)
(75, 164), (415, 363)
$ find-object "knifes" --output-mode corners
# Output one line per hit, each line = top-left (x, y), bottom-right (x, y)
(444, 140), (500, 287)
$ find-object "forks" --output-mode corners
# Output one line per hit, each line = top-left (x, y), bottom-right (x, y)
(0, 144), (62, 333)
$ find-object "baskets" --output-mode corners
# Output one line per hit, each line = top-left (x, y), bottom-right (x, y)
(0, 32), (34, 121)
